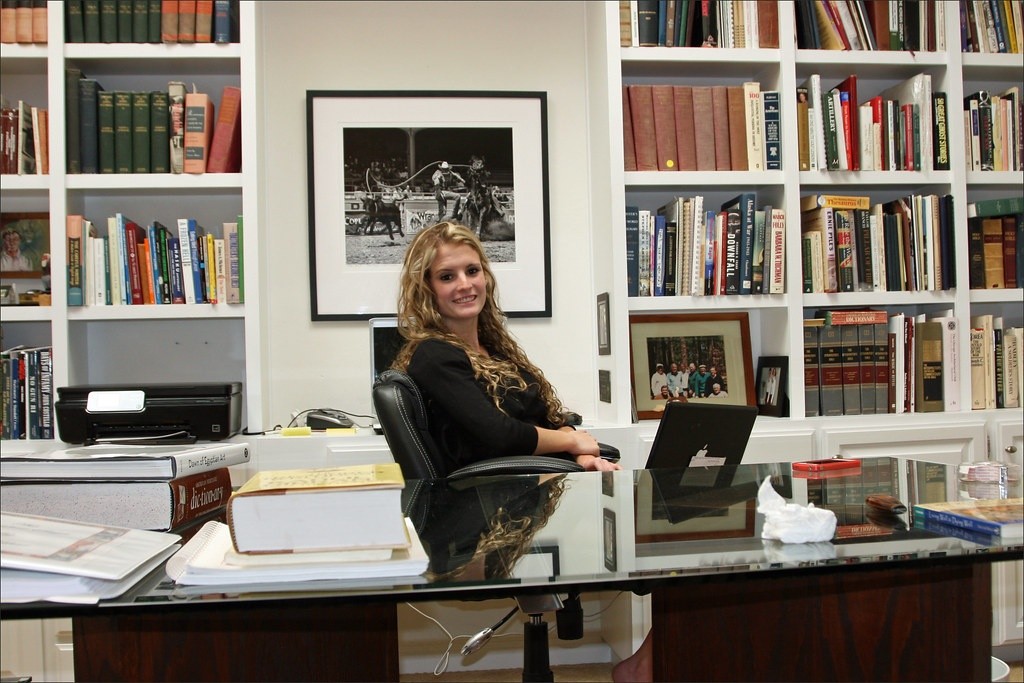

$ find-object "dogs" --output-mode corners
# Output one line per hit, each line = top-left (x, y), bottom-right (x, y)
(360, 194), (408, 240)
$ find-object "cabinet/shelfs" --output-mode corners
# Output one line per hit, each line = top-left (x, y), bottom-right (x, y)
(0, 0), (260, 451)
(584, 0), (1024, 466)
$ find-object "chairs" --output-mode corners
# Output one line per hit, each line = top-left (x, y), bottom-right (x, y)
(373, 376), (586, 683)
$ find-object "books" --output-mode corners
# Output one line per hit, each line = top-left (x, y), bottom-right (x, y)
(913, 498), (1024, 537)
(0, 0), (48, 44)
(622, 82), (782, 171)
(65, 213), (243, 307)
(796, 73), (950, 172)
(800, 193), (956, 294)
(967, 197), (1024, 290)
(0, 345), (54, 439)
(625, 193), (786, 297)
(814, 455), (954, 540)
(0, 442), (430, 602)
(963, 86), (1024, 172)
(970, 315), (1024, 411)
(959, 0), (1024, 54)
(794, 0), (947, 52)
(64, 0), (240, 173)
(0, 100), (49, 175)
(802, 308), (959, 418)
(619, 0), (780, 50)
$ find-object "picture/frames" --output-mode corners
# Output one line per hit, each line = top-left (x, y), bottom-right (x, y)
(305, 90), (552, 321)
(603, 508), (617, 571)
(634, 470), (757, 544)
(0, 210), (50, 278)
(596, 292), (611, 355)
(757, 355), (789, 417)
(509, 547), (560, 580)
(629, 313), (756, 419)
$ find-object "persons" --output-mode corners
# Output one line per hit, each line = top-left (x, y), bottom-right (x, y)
(433, 163), (468, 230)
(765, 462), (785, 489)
(651, 363), (727, 400)
(400, 474), (567, 588)
(764, 369), (778, 406)
(393, 221), (652, 683)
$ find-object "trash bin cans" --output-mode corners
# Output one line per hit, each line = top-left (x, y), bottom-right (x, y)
(991, 656), (1010, 682)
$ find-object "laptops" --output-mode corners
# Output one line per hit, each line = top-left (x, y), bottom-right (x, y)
(649, 462), (770, 526)
(369, 317), (417, 435)
(645, 400), (759, 470)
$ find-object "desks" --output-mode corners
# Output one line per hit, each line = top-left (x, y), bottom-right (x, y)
(0, 449), (1024, 683)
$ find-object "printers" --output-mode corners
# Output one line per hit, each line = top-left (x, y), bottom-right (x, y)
(55, 381), (243, 446)
